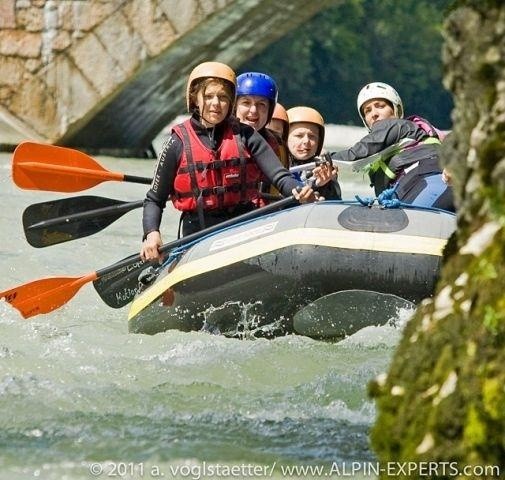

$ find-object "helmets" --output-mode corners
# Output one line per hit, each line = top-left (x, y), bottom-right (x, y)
(235, 72), (278, 123)
(186, 61), (237, 116)
(271, 101), (289, 138)
(287, 106), (325, 157)
(356, 81), (404, 134)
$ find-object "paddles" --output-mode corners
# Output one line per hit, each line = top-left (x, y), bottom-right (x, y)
(13, 141), (154, 193)
(22, 161), (326, 248)
(0, 186), (303, 318)
(93, 248), (182, 308)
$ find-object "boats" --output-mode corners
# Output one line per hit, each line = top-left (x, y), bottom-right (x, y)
(125, 198), (458, 340)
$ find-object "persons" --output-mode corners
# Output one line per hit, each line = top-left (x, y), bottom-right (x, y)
(139, 60), (316, 265)
(319, 82), (458, 210)
(286, 105), (342, 202)
(264, 100), (291, 203)
(233, 71), (339, 207)
